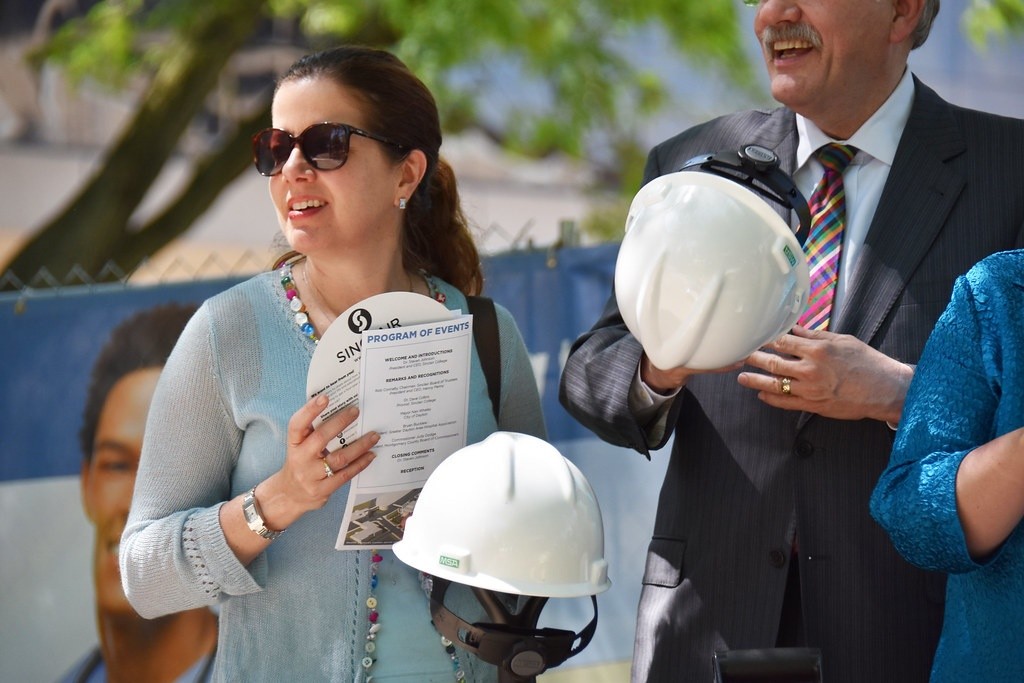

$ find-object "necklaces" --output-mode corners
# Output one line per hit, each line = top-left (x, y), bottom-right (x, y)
(280, 263), (469, 683)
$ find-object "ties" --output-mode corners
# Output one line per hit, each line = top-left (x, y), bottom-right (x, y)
(790, 143), (859, 330)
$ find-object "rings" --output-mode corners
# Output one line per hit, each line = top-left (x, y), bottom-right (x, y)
(322, 457), (334, 478)
(781, 376), (791, 395)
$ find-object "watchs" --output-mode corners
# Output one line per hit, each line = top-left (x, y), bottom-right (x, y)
(242, 483), (287, 539)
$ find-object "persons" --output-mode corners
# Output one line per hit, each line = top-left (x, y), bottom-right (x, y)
(54, 305), (221, 683)
(114, 46), (553, 683)
(867, 247), (1024, 683)
(557, 1), (1024, 683)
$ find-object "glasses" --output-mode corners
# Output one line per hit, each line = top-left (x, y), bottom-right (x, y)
(250, 123), (413, 176)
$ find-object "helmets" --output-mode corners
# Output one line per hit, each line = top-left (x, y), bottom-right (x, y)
(391, 429), (612, 676)
(612, 143), (810, 371)
